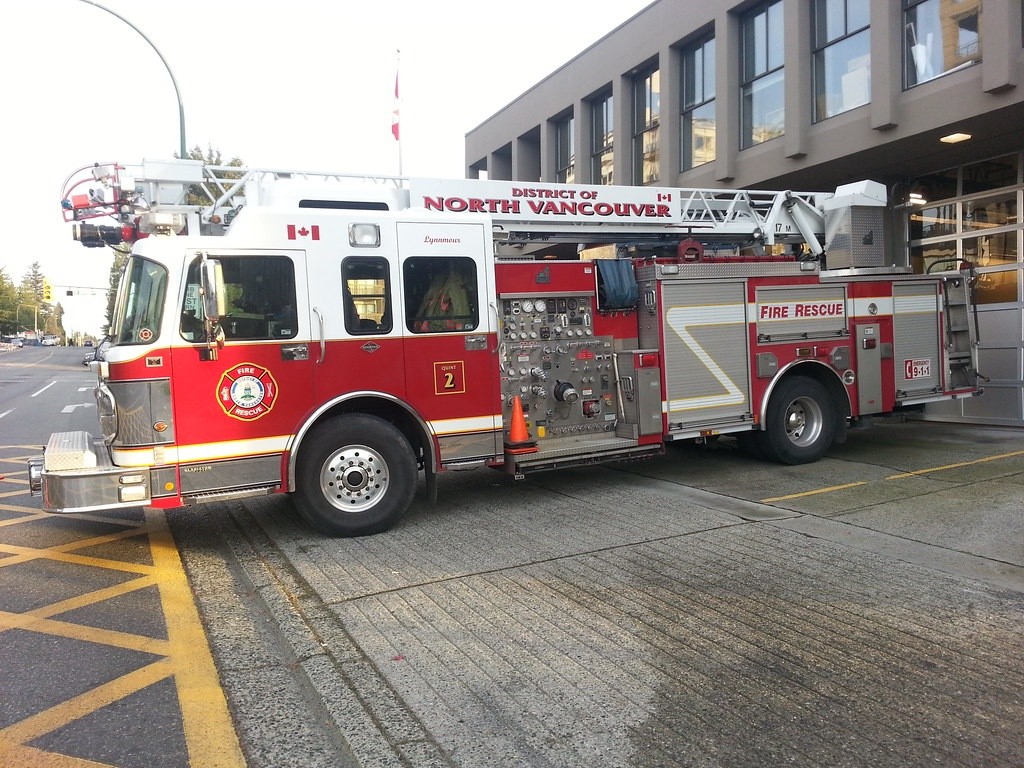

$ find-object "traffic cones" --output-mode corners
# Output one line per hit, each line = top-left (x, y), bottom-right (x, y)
(504, 396), (539, 455)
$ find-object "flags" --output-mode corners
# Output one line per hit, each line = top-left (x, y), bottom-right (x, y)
(391, 70), (400, 141)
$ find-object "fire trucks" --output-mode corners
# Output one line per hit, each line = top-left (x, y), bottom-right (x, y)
(29, 157), (990, 540)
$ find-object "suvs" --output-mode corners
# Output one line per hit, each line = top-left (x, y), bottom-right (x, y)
(7, 339), (23, 348)
(41, 335), (61, 346)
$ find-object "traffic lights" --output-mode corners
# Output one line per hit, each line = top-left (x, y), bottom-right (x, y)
(43, 280), (54, 304)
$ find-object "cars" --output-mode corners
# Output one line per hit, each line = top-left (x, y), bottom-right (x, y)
(84, 341), (92, 346)
(83, 352), (96, 366)
